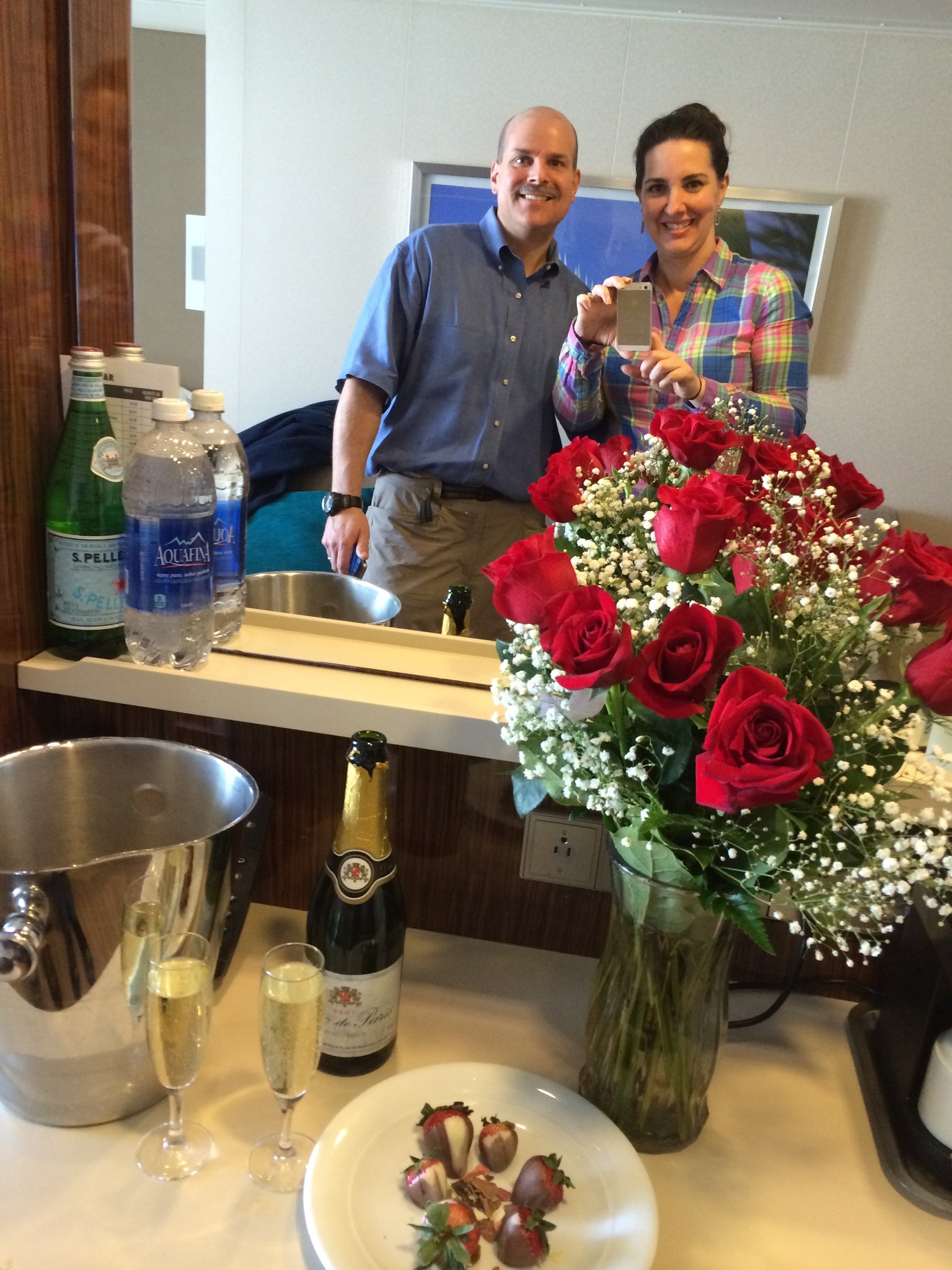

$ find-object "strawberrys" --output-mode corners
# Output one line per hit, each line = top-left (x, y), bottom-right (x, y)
(416, 1101), (474, 1179)
(478, 1116), (518, 1172)
(404, 1155), (447, 1209)
(407, 1198), (481, 1270)
(496, 1206), (557, 1268)
(511, 1153), (576, 1212)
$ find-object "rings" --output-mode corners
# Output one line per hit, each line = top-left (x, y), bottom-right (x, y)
(327, 557), (331, 560)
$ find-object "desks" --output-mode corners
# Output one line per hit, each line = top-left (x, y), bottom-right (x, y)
(0, 900), (952, 1270)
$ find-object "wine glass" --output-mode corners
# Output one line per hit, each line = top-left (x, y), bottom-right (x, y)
(247, 942), (327, 1195)
(133, 931), (217, 1181)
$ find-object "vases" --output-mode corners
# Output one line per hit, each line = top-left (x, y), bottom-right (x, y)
(581, 849), (739, 1157)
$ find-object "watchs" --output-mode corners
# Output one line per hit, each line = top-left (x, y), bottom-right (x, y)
(322, 491), (363, 517)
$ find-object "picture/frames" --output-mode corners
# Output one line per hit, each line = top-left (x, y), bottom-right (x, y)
(410, 159), (846, 367)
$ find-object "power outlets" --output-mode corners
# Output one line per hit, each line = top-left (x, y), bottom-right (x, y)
(519, 812), (616, 894)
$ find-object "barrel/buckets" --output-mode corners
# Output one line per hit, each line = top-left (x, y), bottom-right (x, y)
(245, 571), (402, 626)
(0, 734), (259, 1127)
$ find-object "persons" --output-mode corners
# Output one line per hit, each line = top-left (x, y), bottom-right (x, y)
(321, 106), (591, 643)
(553, 103), (814, 453)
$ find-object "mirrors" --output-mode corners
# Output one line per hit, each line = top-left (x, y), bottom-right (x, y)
(49, 0), (951, 757)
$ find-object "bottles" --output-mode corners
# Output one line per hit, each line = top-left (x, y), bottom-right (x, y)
(112, 341), (144, 365)
(122, 397), (217, 672)
(303, 730), (406, 1078)
(441, 584), (473, 637)
(189, 388), (250, 648)
(43, 346), (133, 662)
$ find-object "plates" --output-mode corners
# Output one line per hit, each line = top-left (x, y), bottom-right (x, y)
(302, 1061), (659, 1269)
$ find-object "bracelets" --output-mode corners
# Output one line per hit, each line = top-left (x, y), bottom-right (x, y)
(687, 376), (702, 400)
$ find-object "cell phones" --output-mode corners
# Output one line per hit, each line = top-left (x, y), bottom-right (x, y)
(617, 282), (652, 351)
(347, 545), (367, 580)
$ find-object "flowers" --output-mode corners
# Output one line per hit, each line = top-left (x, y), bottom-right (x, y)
(478, 388), (952, 1139)
